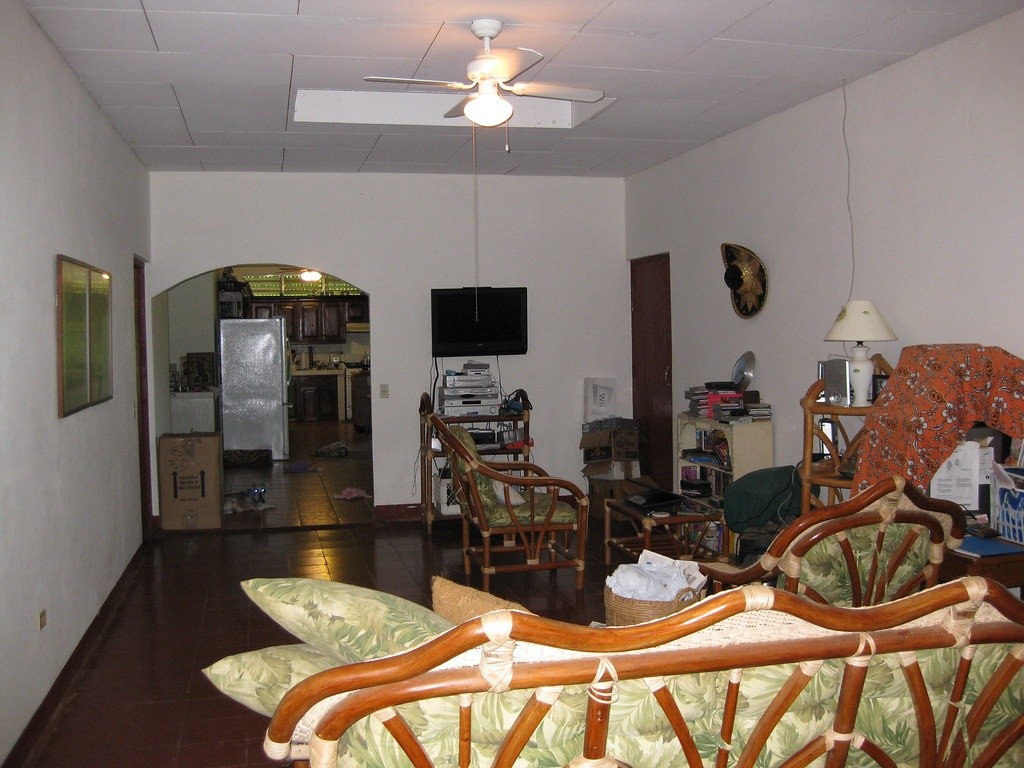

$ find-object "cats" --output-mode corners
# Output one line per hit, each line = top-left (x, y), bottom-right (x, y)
(223, 483), (276, 515)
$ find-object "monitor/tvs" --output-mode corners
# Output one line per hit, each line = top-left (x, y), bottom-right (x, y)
(430, 287), (529, 357)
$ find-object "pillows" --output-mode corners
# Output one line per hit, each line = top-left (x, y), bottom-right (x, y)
(201, 642), (343, 719)
(241, 578), (455, 665)
(491, 478), (527, 506)
(430, 575), (532, 625)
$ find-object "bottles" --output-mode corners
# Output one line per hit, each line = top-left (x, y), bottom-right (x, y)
(838, 456), (858, 480)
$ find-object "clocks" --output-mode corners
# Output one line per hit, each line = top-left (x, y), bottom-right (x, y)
(732, 350), (755, 394)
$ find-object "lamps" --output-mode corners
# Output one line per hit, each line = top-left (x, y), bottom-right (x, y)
(823, 300), (898, 407)
(464, 79), (514, 151)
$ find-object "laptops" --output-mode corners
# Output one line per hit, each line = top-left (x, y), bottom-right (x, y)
(623, 490), (687, 510)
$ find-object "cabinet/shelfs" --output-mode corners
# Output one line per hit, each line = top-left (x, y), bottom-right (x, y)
(676, 414), (773, 556)
(290, 375), (338, 422)
(252, 296), (349, 345)
(800, 354), (894, 516)
(418, 389), (530, 535)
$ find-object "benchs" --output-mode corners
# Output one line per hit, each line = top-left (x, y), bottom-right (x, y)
(263, 576), (1024, 768)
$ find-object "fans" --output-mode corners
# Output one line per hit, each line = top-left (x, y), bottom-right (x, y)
(361, 19), (605, 128)
(274, 266), (322, 282)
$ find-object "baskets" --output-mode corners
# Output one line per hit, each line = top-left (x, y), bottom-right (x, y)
(604, 583), (702, 626)
(990, 504), (1023, 545)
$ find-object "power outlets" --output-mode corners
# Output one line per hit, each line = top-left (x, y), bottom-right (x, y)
(379, 383), (389, 398)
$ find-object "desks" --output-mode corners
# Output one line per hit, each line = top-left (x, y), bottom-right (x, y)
(937, 538), (1024, 604)
(602, 497), (729, 566)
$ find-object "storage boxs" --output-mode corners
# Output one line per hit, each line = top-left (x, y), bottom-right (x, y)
(159, 432), (224, 530)
(579, 418), (659, 521)
(929, 435), (1024, 544)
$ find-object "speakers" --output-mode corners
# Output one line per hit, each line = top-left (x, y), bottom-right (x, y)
(822, 358), (851, 407)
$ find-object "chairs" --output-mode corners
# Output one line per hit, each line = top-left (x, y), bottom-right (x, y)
(427, 413), (589, 592)
(697, 475), (968, 610)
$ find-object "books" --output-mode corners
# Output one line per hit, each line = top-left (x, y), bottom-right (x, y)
(953, 536), (1024, 558)
(683, 381), (771, 421)
(699, 466), (732, 497)
(823, 359), (851, 407)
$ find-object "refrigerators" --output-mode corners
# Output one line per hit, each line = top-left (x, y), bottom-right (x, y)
(218, 316), (294, 460)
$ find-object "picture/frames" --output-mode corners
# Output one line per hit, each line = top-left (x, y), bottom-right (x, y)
(54, 254), (114, 418)
